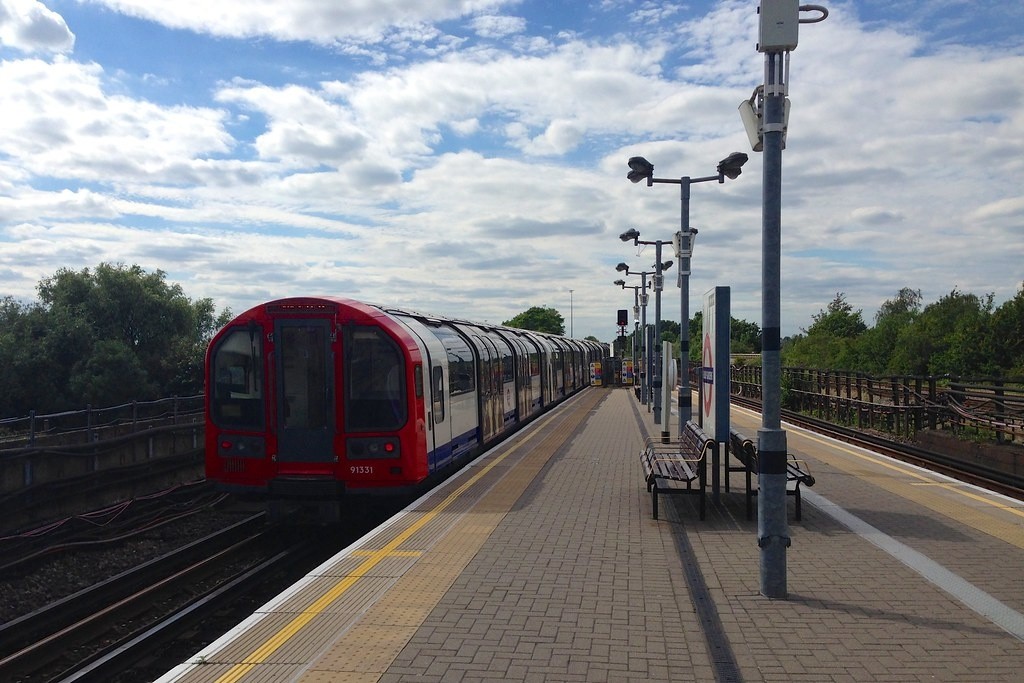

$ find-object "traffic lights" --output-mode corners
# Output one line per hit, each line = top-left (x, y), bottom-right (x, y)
(618, 309), (628, 325)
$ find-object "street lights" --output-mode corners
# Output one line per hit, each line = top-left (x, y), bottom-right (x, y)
(619, 227), (699, 424)
(614, 278), (652, 386)
(569, 289), (574, 337)
(629, 151), (749, 439)
(616, 260), (673, 405)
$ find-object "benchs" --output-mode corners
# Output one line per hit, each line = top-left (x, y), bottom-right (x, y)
(638, 419), (716, 522)
(724, 426), (815, 521)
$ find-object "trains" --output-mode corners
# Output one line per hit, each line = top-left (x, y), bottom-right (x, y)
(202, 292), (613, 532)
